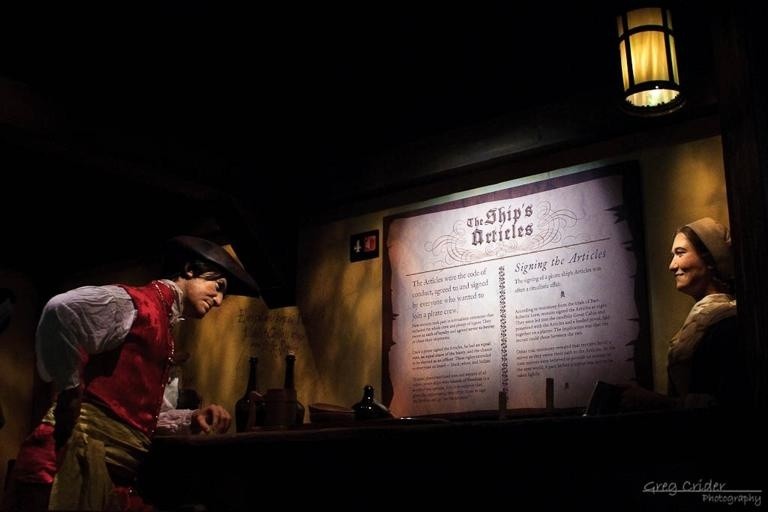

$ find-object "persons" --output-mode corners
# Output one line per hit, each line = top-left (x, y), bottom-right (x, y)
(617, 215), (737, 412)
(2, 230), (265, 511)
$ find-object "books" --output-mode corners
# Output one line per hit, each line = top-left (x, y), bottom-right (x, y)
(583, 380), (623, 416)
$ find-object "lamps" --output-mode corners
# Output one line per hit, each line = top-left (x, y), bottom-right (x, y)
(612, 2), (690, 119)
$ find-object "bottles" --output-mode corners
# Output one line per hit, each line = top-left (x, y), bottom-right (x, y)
(349, 381), (392, 425)
(282, 351), (306, 417)
(233, 357), (265, 430)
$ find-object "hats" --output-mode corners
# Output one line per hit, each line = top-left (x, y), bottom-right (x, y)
(169, 232), (262, 299)
(686, 216), (734, 280)
